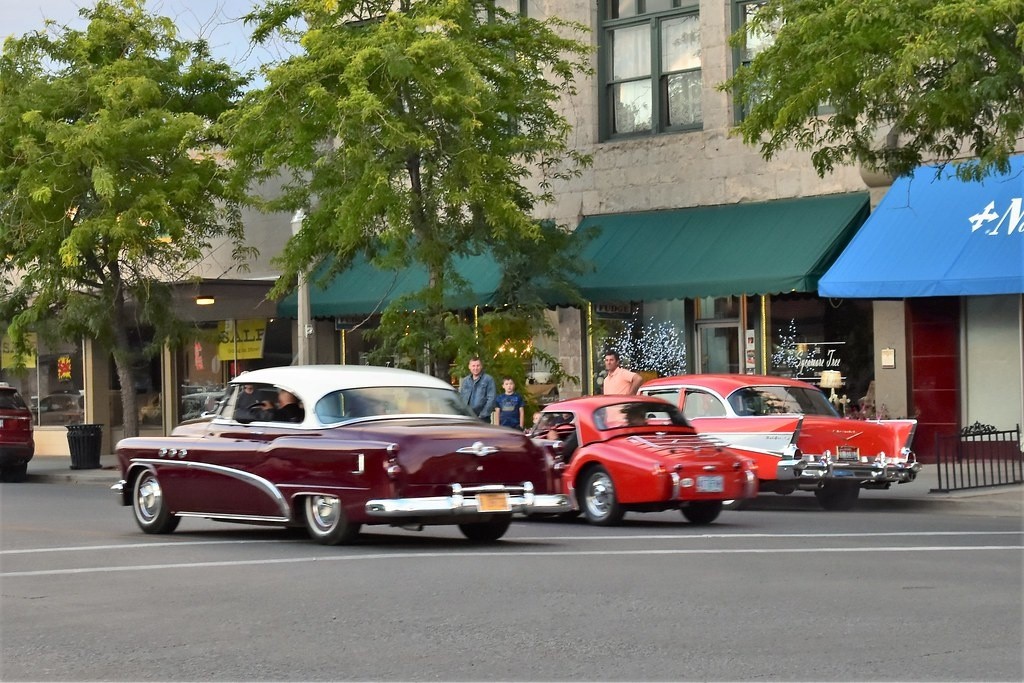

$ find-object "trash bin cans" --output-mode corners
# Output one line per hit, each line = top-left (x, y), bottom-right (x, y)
(65, 423), (104, 470)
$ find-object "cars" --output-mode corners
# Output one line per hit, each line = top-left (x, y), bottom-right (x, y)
(111, 365), (573, 546)
(521, 394), (761, 526)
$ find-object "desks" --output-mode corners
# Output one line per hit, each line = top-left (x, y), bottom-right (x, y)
(64, 412), (84, 424)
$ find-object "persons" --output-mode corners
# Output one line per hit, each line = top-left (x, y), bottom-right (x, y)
(601, 351), (643, 396)
(492, 375), (525, 431)
(547, 421), (580, 491)
(259, 387), (305, 425)
(234, 371), (257, 414)
(458, 357), (497, 428)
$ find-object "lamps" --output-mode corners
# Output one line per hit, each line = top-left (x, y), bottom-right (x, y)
(819, 370), (842, 403)
(196, 296), (215, 305)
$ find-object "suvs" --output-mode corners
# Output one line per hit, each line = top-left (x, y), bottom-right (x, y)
(31, 390), (85, 425)
(0, 382), (35, 479)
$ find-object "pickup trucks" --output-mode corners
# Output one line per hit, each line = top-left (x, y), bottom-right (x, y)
(636, 374), (921, 511)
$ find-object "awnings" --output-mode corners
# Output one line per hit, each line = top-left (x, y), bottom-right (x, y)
(279, 218), (554, 313)
(819, 152), (1024, 299)
(535, 190), (870, 308)
